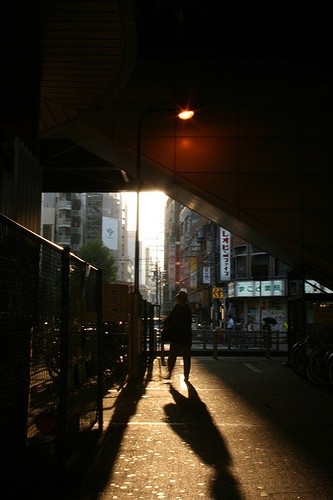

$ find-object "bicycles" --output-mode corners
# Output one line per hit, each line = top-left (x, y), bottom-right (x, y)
(286, 325), (333, 385)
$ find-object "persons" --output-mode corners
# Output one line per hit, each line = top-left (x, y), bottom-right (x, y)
(161, 289), (192, 381)
(226, 314), (272, 351)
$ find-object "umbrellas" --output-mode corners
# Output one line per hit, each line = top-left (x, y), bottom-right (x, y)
(262, 316), (277, 325)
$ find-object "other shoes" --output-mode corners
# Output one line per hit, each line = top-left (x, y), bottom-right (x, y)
(165, 372), (171, 379)
(184, 374), (189, 381)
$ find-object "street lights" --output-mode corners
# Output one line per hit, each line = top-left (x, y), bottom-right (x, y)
(132, 103), (194, 389)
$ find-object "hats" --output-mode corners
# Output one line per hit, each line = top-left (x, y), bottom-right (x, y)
(176, 290), (189, 302)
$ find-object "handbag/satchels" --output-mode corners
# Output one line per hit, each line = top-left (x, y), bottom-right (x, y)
(161, 324), (174, 343)
